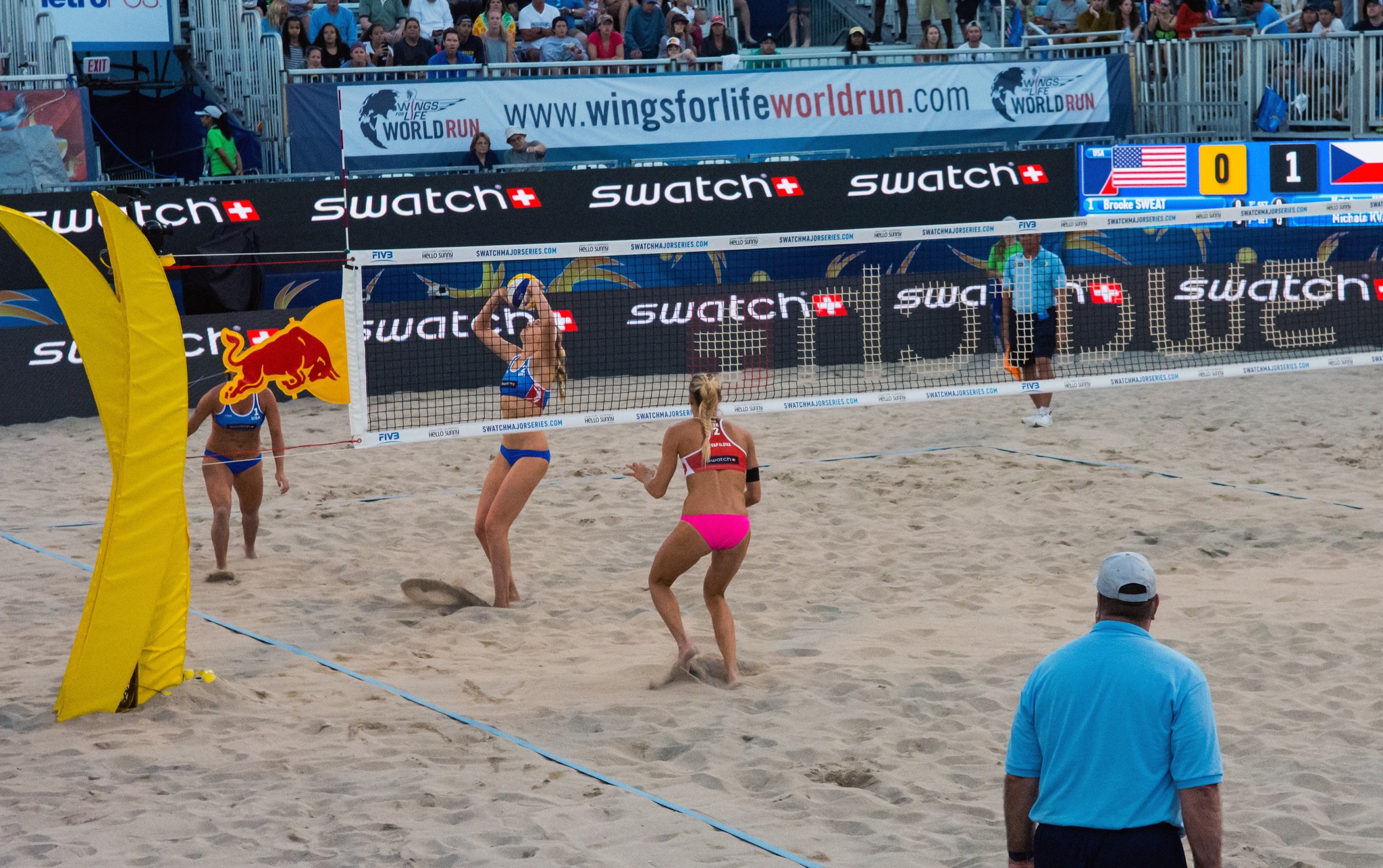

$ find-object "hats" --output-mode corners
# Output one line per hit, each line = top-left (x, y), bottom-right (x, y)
(759, 31), (777, 43)
(1096, 552), (1156, 603)
(459, 15), (472, 24)
(666, 37), (681, 47)
(505, 125), (527, 144)
(644, 0), (658, 5)
(710, 15), (724, 26)
(849, 26), (865, 36)
(1002, 216), (1016, 221)
(194, 105), (222, 119)
(350, 41), (366, 52)
(671, 14), (689, 24)
(966, 21), (982, 41)
(598, 14), (614, 25)
(1302, 4), (1317, 11)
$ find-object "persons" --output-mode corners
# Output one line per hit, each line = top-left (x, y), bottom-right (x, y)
(1001, 232), (1067, 428)
(955, 0), (980, 42)
(459, 132), (506, 174)
(914, 24), (949, 63)
(838, 27), (876, 65)
(1004, 552), (1223, 868)
(916, 0), (954, 49)
(188, 348), (289, 573)
(787, 0), (812, 48)
(503, 126), (547, 172)
(194, 106), (243, 177)
(746, 32), (788, 68)
(622, 373), (761, 684)
(242, 0), (759, 83)
(985, 216), (1024, 355)
(949, 21), (994, 62)
(866, 0), (908, 45)
(473, 279), (568, 609)
(983, 0), (1383, 138)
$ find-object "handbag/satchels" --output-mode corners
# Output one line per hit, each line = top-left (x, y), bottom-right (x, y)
(1257, 86), (1287, 133)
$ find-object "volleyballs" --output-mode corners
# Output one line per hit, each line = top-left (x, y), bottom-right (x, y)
(505, 273), (544, 311)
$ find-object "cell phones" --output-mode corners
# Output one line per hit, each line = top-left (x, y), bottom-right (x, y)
(1154, 0), (1161, 4)
(382, 42), (388, 51)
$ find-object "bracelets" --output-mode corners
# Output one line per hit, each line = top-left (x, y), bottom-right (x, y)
(745, 467), (760, 483)
(1007, 851), (1036, 862)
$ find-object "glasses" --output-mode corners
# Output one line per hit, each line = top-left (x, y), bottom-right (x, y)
(1160, 2), (1169, 7)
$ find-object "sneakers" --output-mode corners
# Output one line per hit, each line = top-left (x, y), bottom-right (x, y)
(867, 38), (883, 45)
(1021, 408), (1053, 429)
(894, 38), (911, 45)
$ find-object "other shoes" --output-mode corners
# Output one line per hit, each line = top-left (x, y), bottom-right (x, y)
(1332, 109), (1342, 122)
